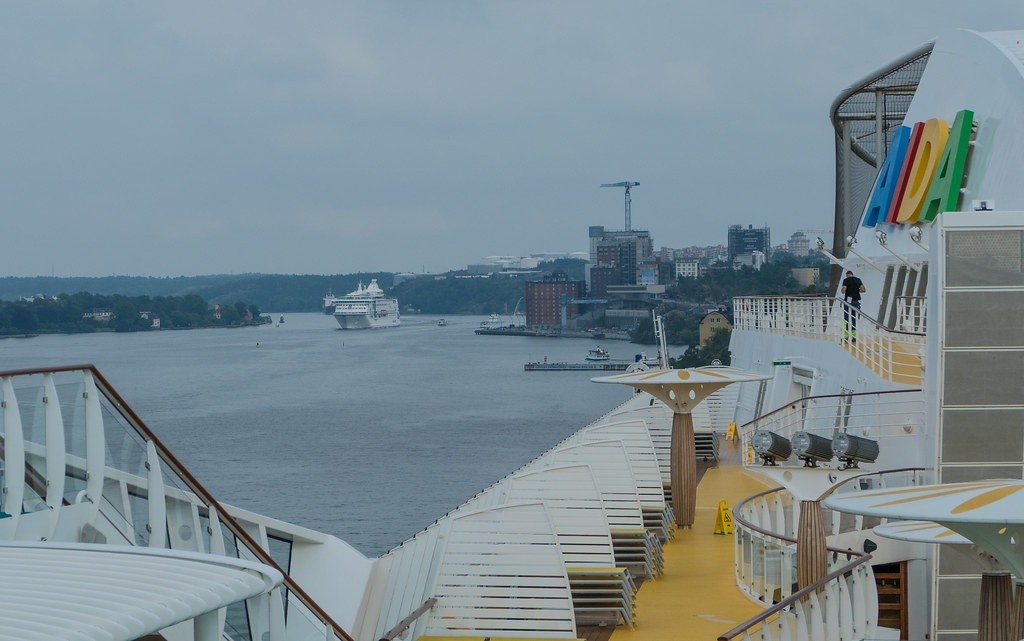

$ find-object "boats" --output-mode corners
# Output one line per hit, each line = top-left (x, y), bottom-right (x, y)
(585, 343), (611, 360)
(321, 278), (402, 329)
(489, 311), (501, 322)
(437, 318), (447, 326)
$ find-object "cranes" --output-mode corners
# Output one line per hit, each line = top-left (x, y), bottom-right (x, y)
(599, 181), (641, 232)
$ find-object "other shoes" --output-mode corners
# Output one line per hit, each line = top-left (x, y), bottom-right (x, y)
(852, 338), (856, 342)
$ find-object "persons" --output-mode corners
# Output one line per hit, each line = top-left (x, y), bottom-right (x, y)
(840, 270), (866, 343)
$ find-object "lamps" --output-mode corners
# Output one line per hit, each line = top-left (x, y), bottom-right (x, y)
(832, 434), (879, 469)
(791, 432), (833, 468)
(875, 229), (920, 274)
(910, 226), (929, 252)
(846, 236), (886, 273)
(751, 431), (790, 466)
(815, 237), (849, 271)
